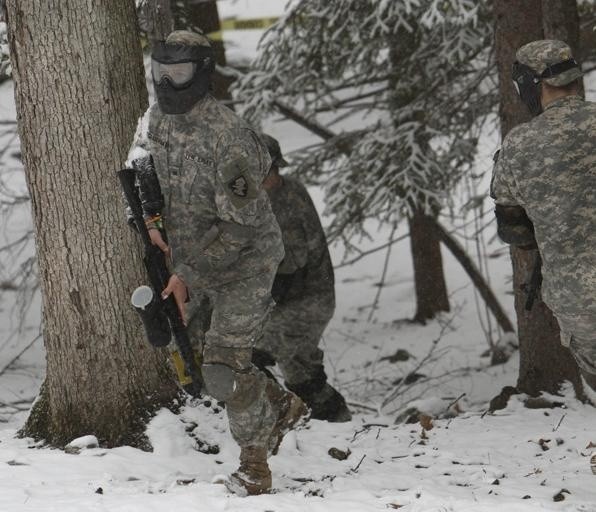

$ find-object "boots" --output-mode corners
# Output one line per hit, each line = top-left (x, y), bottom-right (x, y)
(223, 440), (275, 499)
(264, 377), (309, 457)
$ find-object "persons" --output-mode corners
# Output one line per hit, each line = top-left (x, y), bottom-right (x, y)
(119, 30), (311, 494)
(249, 134), (355, 424)
(492, 41), (595, 394)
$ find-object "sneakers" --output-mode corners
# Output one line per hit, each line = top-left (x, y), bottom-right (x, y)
(308, 388), (354, 424)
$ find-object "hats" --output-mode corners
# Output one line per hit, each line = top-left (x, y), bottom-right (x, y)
(167, 30), (213, 65)
(254, 131), (292, 169)
(513, 37), (587, 90)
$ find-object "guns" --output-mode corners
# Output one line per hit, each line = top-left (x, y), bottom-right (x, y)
(118, 155), (204, 393)
